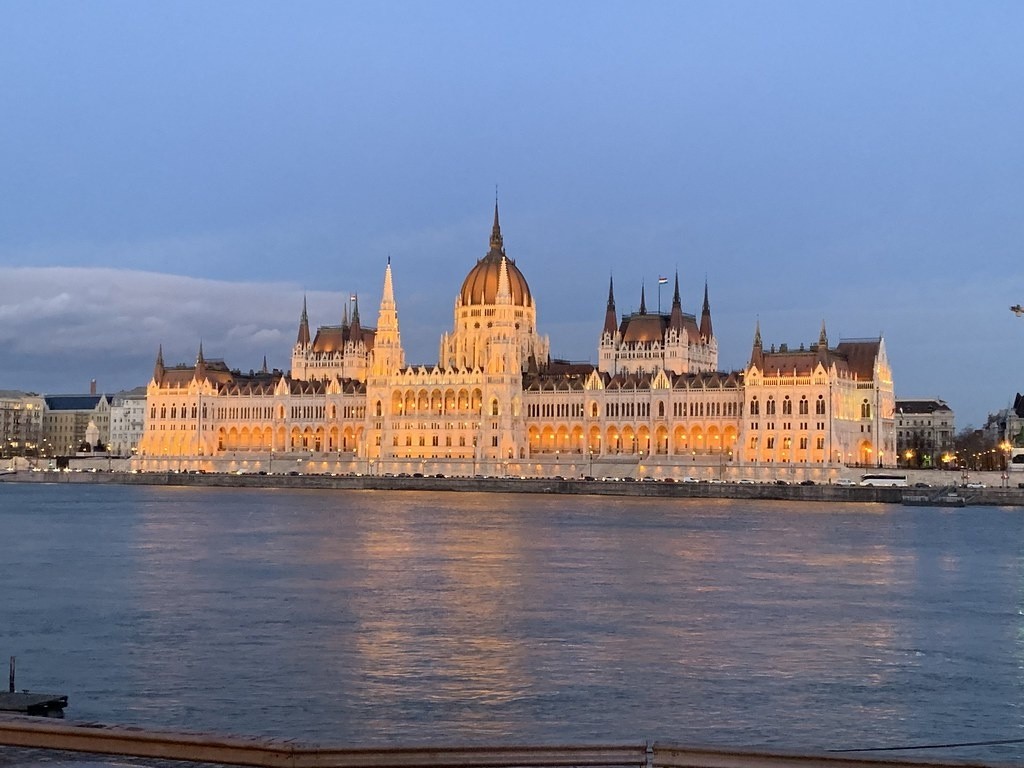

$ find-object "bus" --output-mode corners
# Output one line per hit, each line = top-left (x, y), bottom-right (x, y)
(859, 474), (908, 489)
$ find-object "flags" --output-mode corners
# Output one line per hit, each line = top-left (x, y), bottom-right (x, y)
(658, 278), (667, 284)
(349, 296), (358, 302)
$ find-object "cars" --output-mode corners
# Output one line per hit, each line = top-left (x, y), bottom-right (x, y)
(966, 482), (987, 489)
(774, 480), (791, 485)
(5, 466), (272, 475)
(797, 479), (815, 486)
(710, 478), (726, 484)
(601, 476), (619, 483)
(664, 477), (674, 482)
(584, 476), (597, 482)
(555, 475), (568, 480)
(382, 471), (445, 478)
(322, 471), (336, 476)
(643, 477), (658, 483)
(836, 479), (857, 488)
(621, 476), (636, 482)
(473, 474), (489, 479)
(683, 477), (699, 483)
(348, 471), (362, 477)
(916, 482), (932, 488)
(502, 474), (514, 479)
(287, 471), (303, 475)
(737, 479), (755, 484)
